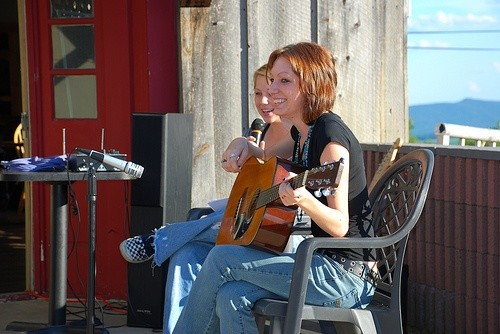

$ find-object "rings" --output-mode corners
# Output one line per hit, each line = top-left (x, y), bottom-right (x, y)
(230, 153), (237, 157)
(222, 159), (228, 163)
(281, 195), (286, 199)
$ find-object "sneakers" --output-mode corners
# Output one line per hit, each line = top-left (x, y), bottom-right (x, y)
(120, 231), (156, 263)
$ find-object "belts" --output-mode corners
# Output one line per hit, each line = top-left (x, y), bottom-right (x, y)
(317, 248), (380, 288)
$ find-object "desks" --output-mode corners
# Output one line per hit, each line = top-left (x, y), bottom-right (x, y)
(0, 169), (137, 334)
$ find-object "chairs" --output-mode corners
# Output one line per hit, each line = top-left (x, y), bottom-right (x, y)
(254, 149), (435, 334)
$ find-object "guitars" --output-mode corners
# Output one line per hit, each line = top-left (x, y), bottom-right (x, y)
(217, 156), (345, 253)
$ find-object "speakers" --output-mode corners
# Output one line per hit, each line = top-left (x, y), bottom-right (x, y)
(126, 113), (194, 329)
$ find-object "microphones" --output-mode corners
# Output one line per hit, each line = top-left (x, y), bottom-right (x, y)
(251, 118), (265, 147)
(75, 148), (144, 178)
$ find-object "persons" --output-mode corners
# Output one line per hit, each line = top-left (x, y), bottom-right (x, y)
(170, 41), (379, 334)
(119, 63), (292, 334)
